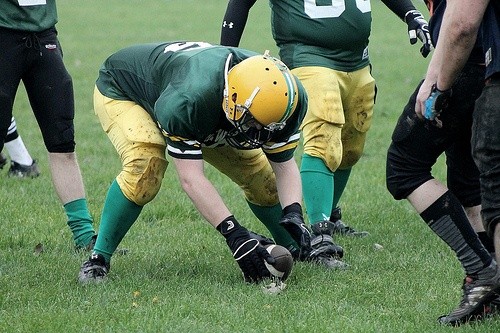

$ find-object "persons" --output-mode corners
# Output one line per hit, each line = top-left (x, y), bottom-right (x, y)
(78, 41), (308, 296)
(220, 0), (500, 325)
(0, 0), (129, 256)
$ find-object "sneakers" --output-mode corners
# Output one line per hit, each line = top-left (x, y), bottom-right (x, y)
(440, 261), (500, 325)
(80, 255), (109, 285)
(331, 206), (371, 238)
(308, 221), (344, 258)
(8, 160), (40, 178)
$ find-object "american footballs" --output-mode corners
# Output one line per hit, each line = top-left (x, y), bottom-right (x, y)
(241, 244), (294, 282)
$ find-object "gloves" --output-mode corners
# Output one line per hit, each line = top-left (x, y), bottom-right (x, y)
(422, 84), (453, 129)
(405, 8), (430, 59)
(279, 204), (312, 262)
(214, 217), (275, 287)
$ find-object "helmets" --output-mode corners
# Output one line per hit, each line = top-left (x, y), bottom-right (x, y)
(222, 52), (298, 143)
